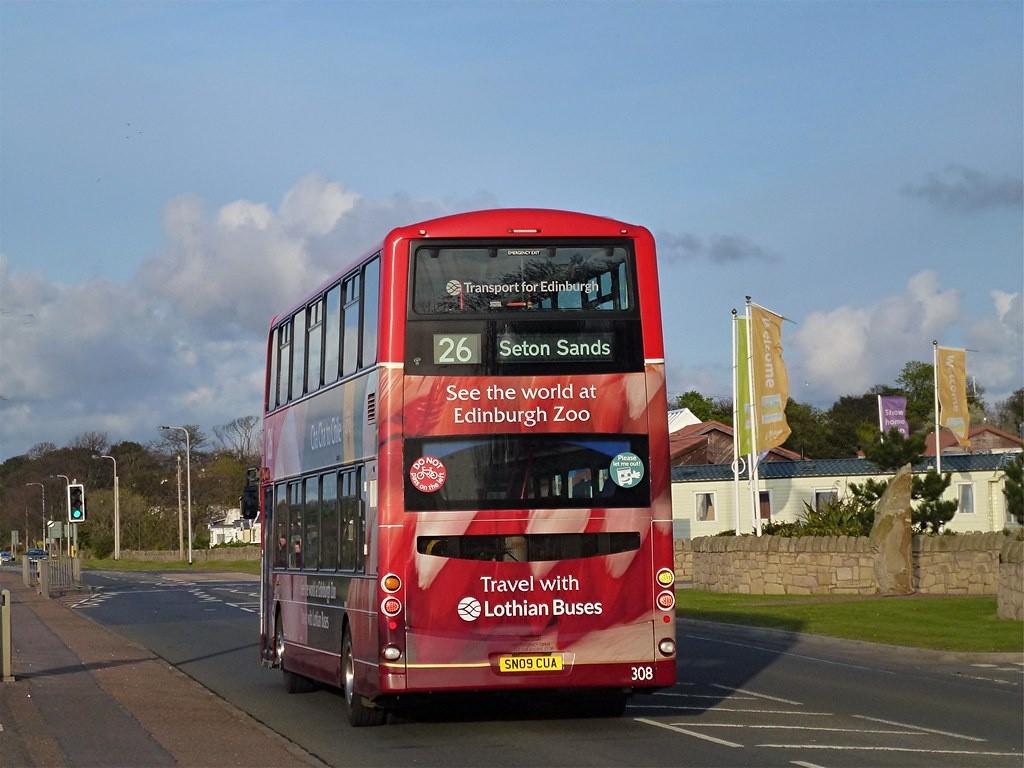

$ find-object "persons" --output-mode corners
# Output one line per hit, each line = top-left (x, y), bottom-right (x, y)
(279, 534), (287, 566)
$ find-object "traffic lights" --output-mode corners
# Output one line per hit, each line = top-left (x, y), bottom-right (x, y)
(66, 484), (86, 522)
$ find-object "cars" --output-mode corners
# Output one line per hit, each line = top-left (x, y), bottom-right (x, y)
(0, 552), (12, 563)
(24, 548), (48, 565)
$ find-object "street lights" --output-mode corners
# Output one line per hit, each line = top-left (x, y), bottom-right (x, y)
(56, 475), (72, 557)
(26, 482), (46, 552)
(175, 454), (185, 561)
(91, 454), (117, 561)
(156, 425), (193, 565)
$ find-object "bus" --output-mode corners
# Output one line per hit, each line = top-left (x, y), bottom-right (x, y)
(241, 206), (677, 725)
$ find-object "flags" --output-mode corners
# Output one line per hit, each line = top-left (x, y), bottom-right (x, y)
(737, 319), (757, 457)
(937, 348), (971, 447)
(881, 397), (909, 439)
(750, 304), (792, 450)
